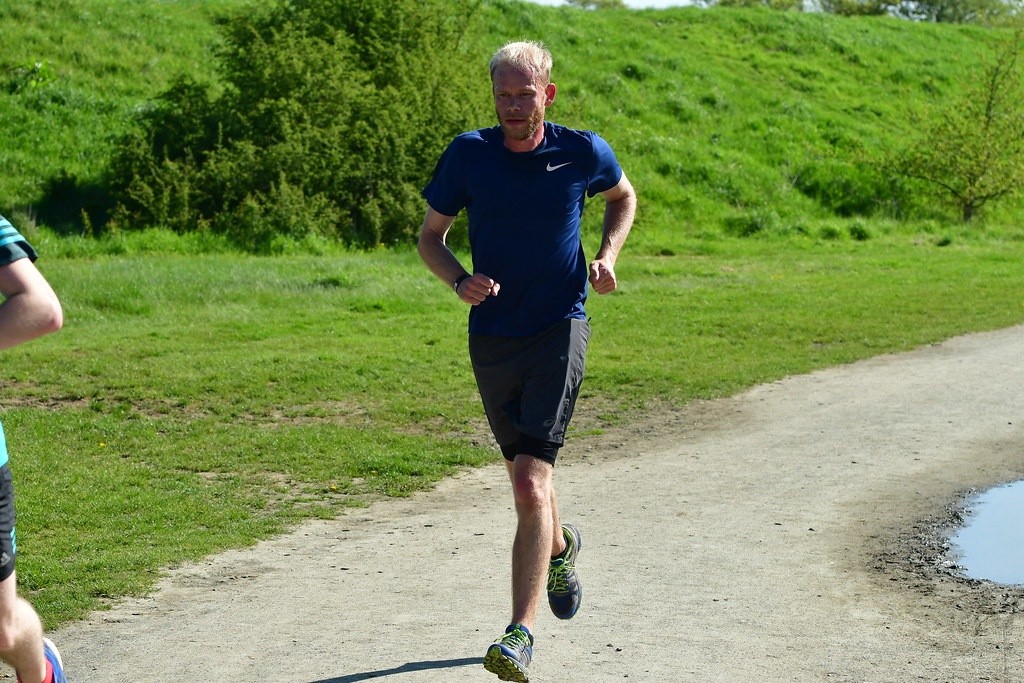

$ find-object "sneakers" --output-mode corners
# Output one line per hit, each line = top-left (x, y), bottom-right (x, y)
(484, 624), (534, 683)
(548, 524), (582, 621)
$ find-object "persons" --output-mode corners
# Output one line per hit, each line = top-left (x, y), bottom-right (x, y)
(0, 211), (67, 683)
(419, 42), (638, 683)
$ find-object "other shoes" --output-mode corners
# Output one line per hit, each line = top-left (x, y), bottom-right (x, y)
(16, 637), (68, 683)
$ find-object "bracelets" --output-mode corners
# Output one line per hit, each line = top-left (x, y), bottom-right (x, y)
(452, 272), (472, 294)
